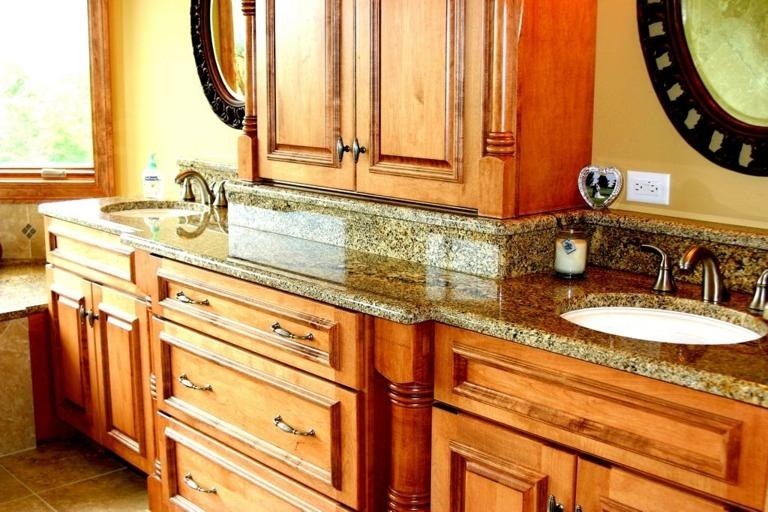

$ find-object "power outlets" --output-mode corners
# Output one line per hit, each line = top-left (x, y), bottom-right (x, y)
(626, 171), (671, 203)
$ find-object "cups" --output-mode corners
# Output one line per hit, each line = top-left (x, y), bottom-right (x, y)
(554, 225), (588, 280)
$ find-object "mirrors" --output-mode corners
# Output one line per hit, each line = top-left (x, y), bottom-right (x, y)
(635, 0), (767, 176)
(189, 0), (246, 131)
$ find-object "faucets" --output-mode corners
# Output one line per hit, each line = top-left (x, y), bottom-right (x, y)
(175, 211), (212, 239)
(679, 246), (723, 301)
(175, 171), (215, 204)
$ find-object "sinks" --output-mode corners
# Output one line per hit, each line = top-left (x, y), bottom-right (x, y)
(100, 201), (212, 220)
(555, 292), (768, 345)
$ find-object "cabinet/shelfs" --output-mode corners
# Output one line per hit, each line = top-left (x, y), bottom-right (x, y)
(431, 326), (768, 512)
(150, 256), (428, 511)
(240, 1), (596, 221)
(47, 216), (151, 480)
